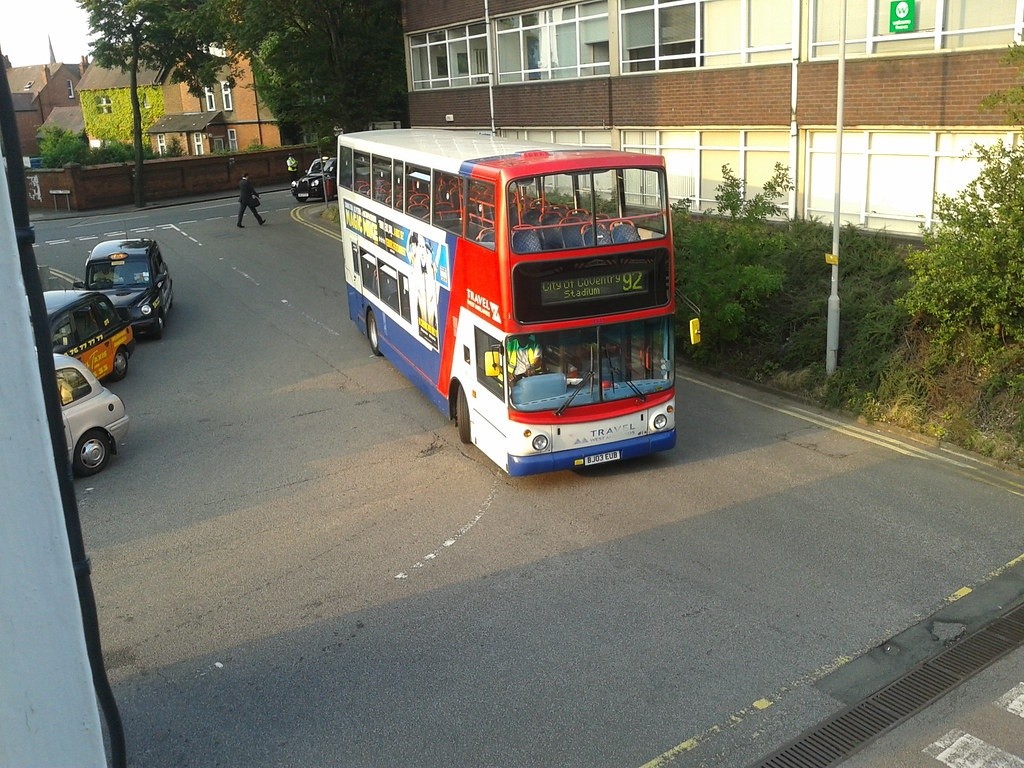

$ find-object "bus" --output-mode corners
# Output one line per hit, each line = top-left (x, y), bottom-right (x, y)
(336, 127), (700, 475)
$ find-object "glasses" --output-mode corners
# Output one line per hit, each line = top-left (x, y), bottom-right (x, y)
(289, 155), (292, 156)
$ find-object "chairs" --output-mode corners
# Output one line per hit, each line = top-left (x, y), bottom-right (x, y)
(350, 175), (641, 252)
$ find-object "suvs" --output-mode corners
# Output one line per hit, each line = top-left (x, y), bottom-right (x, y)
(291, 157), (338, 204)
(71, 222), (174, 343)
(27, 289), (137, 393)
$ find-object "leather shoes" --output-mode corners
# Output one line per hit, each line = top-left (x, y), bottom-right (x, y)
(260, 219), (266, 225)
(237, 224), (244, 228)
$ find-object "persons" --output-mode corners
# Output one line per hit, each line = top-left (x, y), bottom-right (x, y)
(55, 377), (77, 402)
(506, 333), (543, 387)
(237, 172), (267, 228)
(93, 264), (125, 284)
(286, 151), (299, 186)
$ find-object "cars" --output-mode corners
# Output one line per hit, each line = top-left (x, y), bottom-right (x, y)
(53, 354), (133, 479)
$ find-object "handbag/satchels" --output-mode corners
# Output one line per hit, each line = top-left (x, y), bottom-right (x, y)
(253, 198), (260, 207)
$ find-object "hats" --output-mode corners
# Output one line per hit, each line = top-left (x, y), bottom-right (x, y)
(289, 153), (293, 155)
